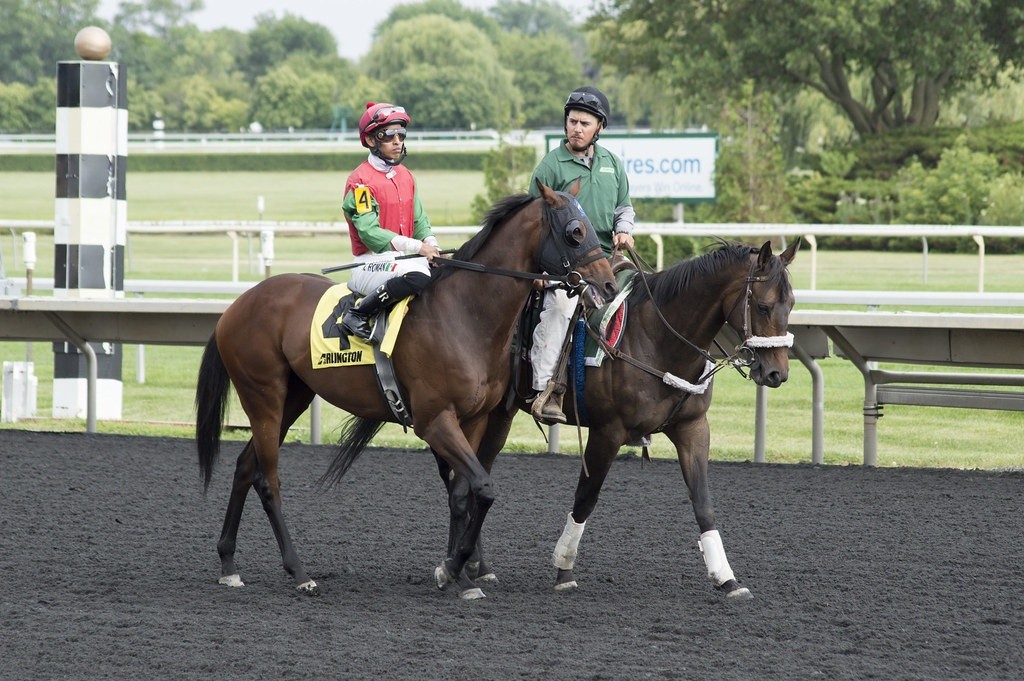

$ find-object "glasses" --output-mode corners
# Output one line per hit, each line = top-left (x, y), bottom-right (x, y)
(371, 128), (407, 142)
(363, 106), (406, 135)
(567, 91), (602, 110)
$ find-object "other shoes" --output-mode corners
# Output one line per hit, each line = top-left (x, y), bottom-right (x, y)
(532, 392), (567, 423)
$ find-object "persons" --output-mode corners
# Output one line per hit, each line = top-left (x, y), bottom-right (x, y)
(341, 101), (450, 346)
(525, 86), (635, 424)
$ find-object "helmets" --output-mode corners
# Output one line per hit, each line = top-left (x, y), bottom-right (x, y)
(359, 102), (412, 148)
(564, 86), (610, 129)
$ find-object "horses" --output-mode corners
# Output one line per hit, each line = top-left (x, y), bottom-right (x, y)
(189, 175), (803, 606)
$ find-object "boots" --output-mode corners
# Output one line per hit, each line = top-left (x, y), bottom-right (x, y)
(341, 280), (399, 338)
